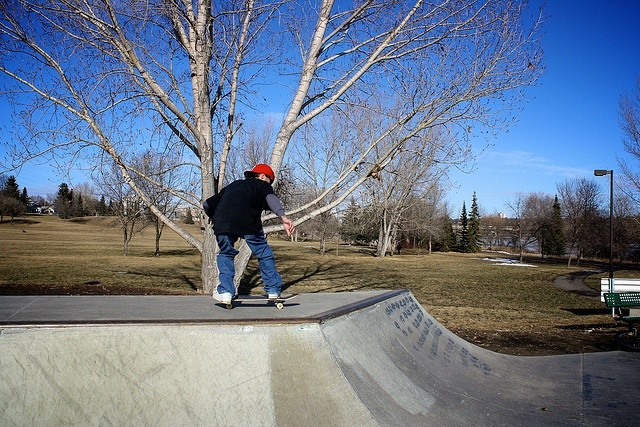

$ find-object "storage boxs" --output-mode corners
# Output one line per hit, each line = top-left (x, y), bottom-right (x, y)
(605, 292), (640, 307)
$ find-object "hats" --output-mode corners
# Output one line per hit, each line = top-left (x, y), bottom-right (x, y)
(244, 164), (274, 184)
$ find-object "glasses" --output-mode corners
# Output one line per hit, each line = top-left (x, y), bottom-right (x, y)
(269, 177), (273, 184)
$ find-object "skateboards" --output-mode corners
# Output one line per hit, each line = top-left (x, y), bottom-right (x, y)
(209, 292), (302, 311)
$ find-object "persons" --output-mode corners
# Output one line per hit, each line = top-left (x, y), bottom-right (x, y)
(203, 163), (295, 304)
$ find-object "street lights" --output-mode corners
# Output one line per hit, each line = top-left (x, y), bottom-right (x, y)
(595, 170), (614, 278)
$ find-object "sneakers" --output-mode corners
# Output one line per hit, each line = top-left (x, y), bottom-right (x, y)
(268, 294), (279, 299)
(213, 292), (233, 304)
